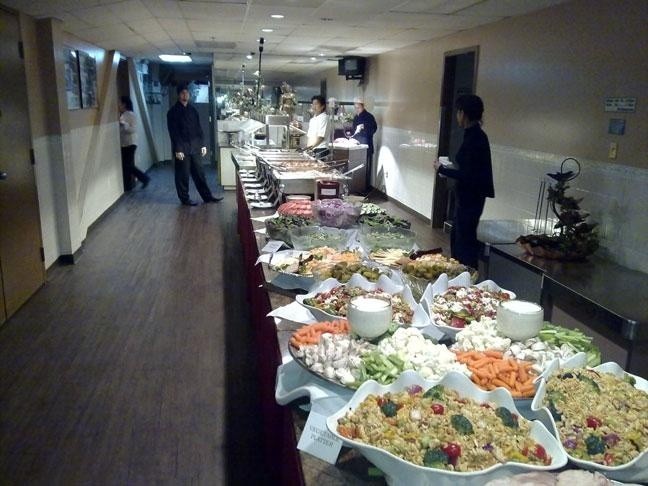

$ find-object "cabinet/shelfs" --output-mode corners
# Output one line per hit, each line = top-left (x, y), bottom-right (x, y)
(216, 140), (237, 191)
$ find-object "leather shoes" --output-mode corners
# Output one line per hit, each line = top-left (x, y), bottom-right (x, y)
(206, 195), (223, 204)
(181, 198), (199, 206)
(140, 176), (151, 190)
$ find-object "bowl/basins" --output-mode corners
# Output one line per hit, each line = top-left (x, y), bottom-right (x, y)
(346, 295), (394, 341)
(496, 299), (544, 341)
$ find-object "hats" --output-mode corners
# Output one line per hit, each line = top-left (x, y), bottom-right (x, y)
(352, 96), (365, 104)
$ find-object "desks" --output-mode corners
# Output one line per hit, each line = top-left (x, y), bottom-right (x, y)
(230, 147), (648, 486)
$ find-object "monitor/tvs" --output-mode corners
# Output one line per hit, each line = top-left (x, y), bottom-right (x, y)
(338, 58), (364, 76)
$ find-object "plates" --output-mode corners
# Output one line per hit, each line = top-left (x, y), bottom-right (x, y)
(446, 331), (603, 408)
(268, 249), (391, 291)
(363, 249), (417, 276)
(266, 272), (445, 341)
(326, 369), (569, 486)
(419, 270), (516, 340)
(286, 328), (472, 391)
(530, 360), (648, 472)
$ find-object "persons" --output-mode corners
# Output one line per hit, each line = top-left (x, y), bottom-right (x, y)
(433, 94), (495, 271)
(167, 85), (224, 206)
(118, 95), (151, 193)
(350, 96), (377, 196)
(305, 95), (332, 161)
